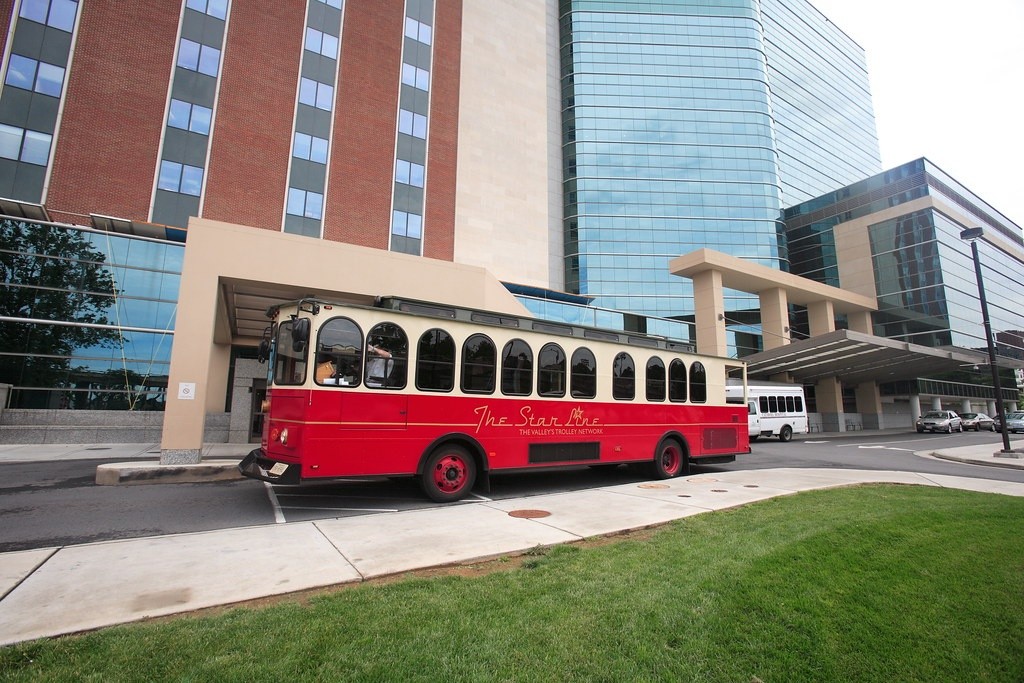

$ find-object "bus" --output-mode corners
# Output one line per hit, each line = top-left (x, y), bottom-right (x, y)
(236, 294), (752, 506)
(725, 377), (810, 442)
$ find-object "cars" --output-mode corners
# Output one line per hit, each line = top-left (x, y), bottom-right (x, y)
(915, 410), (963, 434)
(958, 412), (995, 432)
(993, 410), (1024, 434)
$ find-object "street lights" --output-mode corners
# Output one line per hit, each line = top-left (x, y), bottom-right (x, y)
(960, 227), (1019, 459)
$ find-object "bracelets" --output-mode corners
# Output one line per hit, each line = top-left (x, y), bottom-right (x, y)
(371, 347), (374, 352)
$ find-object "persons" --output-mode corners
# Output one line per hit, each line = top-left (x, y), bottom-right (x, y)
(364, 344), (395, 387)
